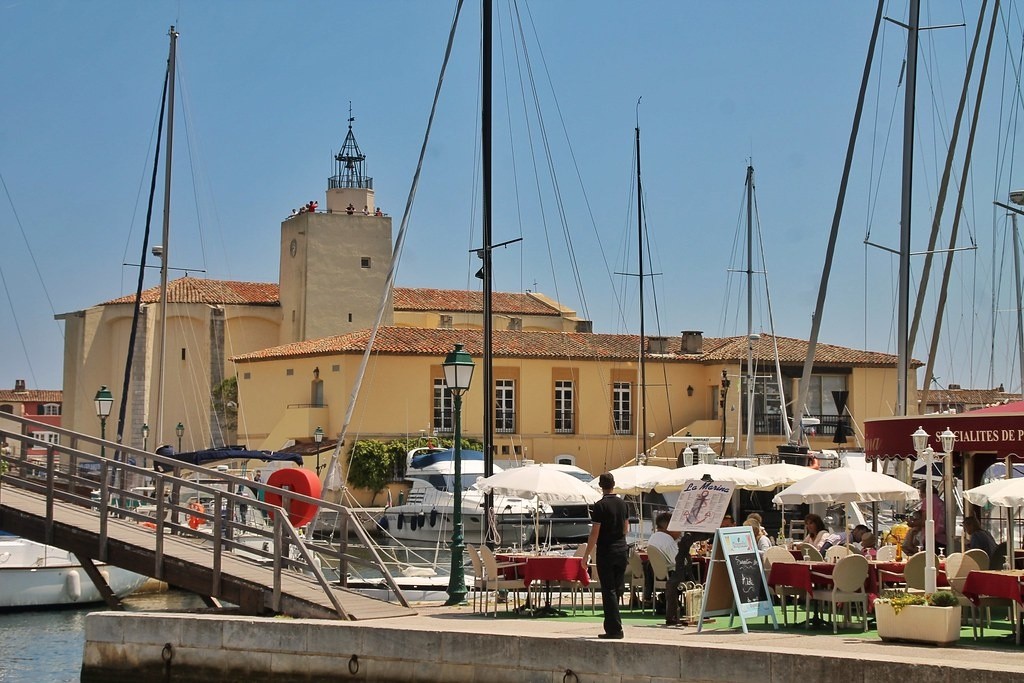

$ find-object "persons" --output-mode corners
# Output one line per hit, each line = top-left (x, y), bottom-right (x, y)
(647, 514), (698, 616)
(742, 518), (773, 561)
(581, 471), (630, 640)
(376, 207), (382, 216)
(345, 203), (356, 214)
(362, 205), (370, 216)
(747, 513), (768, 535)
(961, 517), (999, 559)
(290, 201), (318, 219)
(905, 480), (948, 558)
(819, 524), (872, 559)
(707, 514), (738, 544)
(796, 513), (831, 552)
(860, 532), (880, 560)
(899, 511), (924, 556)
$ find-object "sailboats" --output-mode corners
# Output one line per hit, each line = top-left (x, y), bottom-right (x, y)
(91, 23), (304, 611)
(606, 0), (1023, 553)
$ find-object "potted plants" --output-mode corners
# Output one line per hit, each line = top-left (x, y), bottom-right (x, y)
(875, 590), (962, 647)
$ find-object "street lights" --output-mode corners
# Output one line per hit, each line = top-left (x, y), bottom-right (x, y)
(911, 425), (957, 593)
(441, 343), (476, 606)
(314, 426), (324, 476)
(141, 423), (150, 468)
(93, 384), (115, 457)
(175, 421), (185, 454)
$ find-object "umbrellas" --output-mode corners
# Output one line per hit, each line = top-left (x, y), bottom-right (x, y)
(469, 456), (1024, 565)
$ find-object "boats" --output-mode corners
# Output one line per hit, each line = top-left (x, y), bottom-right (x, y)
(377, 441), (554, 547)
(510, 460), (603, 541)
(0, 532), (148, 611)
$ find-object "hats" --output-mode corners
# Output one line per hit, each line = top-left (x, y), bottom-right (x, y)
(861, 532), (876, 546)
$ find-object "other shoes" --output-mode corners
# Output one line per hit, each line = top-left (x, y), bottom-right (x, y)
(598, 633), (623, 639)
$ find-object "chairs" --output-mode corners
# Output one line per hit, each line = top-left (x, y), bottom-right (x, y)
(466, 536), (1024, 644)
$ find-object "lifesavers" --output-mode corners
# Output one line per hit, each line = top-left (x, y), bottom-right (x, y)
(185, 502), (205, 528)
(806, 451), (821, 470)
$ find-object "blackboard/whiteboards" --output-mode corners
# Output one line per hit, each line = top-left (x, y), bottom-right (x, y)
(699, 526), (774, 616)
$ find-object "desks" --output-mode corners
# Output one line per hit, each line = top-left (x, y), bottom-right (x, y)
(767, 560), (949, 629)
(495, 553), (589, 618)
(962, 569), (1024, 644)
